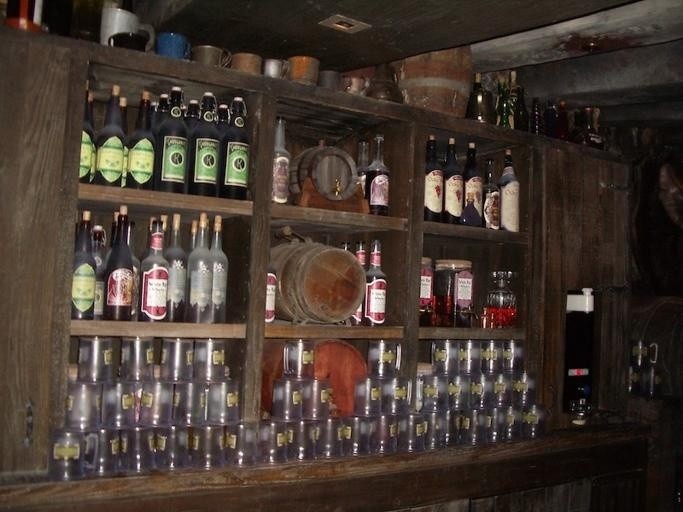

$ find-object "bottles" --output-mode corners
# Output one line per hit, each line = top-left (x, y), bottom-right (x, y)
(498, 146), (521, 232)
(470, 68), (606, 148)
(417, 254), (435, 326)
(460, 192), (483, 226)
(366, 129), (391, 216)
(71, 205), (230, 324)
(434, 257), (474, 325)
(425, 134), (444, 222)
(444, 136), (464, 224)
(355, 138), (371, 194)
(273, 115), (293, 204)
(263, 248), (278, 324)
(79, 82), (253, 200)
(349, 241), (369, 327)
(464, 142), (484, 217)
(365, 239), (388, 328)
(482, 158), (501, 229)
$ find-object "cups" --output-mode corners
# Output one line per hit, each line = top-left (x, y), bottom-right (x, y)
(449, 411), (465, 447)
(108, 32), (147, 52)
(514, 373), (537, 409)
(343, 75), (368, 94)
(482, 339), (504, 376)
(353, 376), (382, 416)
(48, 432), (86, 481)
(282, 339), (314, 379)
(421, 375), (449, 412)
(346, 417), (371, 459)
(191, 426), (227, 473)
(288, 56), (320, 87)
(156, 426), (191, 472)
(231, 53), (262, 76)
(483, 407), (503, 444)
(194, 339), (226, 384)
(398, 414), (427, 454)
(471, 375), (494, 410)
(382, 376), (408, 417)
(76, 335), (114, 385)
(366, 339), (396, 379)
(319, 71), (352, 91)
(120, 337), (155, 385)
(100, 7), (155, 51)
(458, 339), (482, 376)
(504, 339), (526, 374)
(226, 422), (259, 468)
(431, 339), (458, 377)
(372, 416), (398, 456)
(175, 383), (206, 428)
(121, 428), (156, 474)
(65, 382), (100, 434)
(449, 377), (471, 411)
(524, 404), (546, 440)
(156, 32), (191, 60)
(206, 379), (240, 426)
(270, 379), (302, 421)
(192, 45), (232, 69)
(302, 379), (330, 420)
(138, 382), (174, 428)
(422, 410), (447, 450)
(464, 409), (485, 446)
(495, 374), (512, 409)
(257, 422), (288, 465)
(160, 338), (194, 384)
(87, 430), (120, 479)
(262, 59), (290, 79)
(501, 406), (522, 444)
(101, 382), (137, 430)
(317, 415), (346, 458)
(287, 420), (317, 463)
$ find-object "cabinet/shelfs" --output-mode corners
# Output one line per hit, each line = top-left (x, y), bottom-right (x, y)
(51, 40), (661, 512)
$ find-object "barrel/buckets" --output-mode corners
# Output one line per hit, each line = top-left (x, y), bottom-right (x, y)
(263, 338), (367, 419)
(269, 241), (367, 325)
(400, 44), (474, 119)
(289, 145), (358, 201)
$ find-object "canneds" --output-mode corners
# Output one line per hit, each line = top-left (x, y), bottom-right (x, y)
(258, 338), (344, 460)
(344, 340), (425, 456)
(47, 334), (257, 484)
(420, 339), (541, 448)
